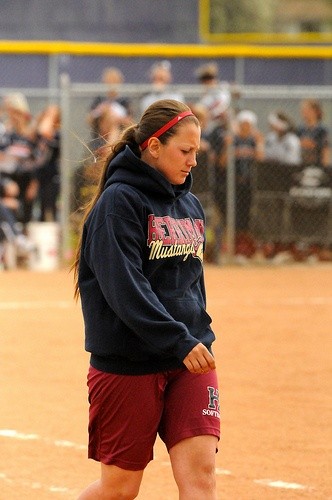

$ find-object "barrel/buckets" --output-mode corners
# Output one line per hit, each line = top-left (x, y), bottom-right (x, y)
(26, 222), (62, 270)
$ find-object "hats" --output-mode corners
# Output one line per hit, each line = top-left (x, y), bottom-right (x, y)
(237, 111), (257, 127)
(199, 64), (216, 80)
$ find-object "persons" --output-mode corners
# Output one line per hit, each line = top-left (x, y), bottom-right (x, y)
(73, 99), (221, 499)
(0, 61), (332, 270)
